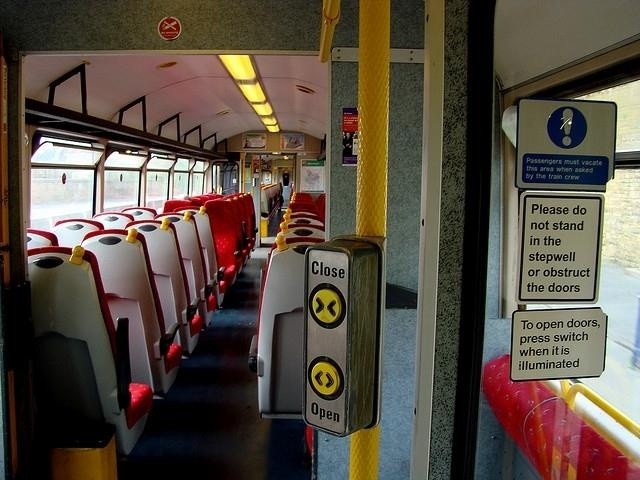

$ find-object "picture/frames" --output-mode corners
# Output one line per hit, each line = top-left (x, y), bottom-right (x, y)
(242, 132), (267, 151)
(280, 133), (304, 150)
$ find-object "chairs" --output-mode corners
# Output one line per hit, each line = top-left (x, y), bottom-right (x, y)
(279, 214), (325, 226)
(185, 193), (252, 204)
(204, 200), (243, 276)
(78, 227), (182, 395)
(27, 246), (152, 459)
(286, 190), (328, 210)
(173, 206), (235, 310)
(92, 212), (133, 230)
(124, 220), (202, 359)
(161, 200), (194, 212)
(282, 211), (322, 218)
(265, 223), (325, 265)
(25, 227), (59, 251)
(248, 233), (328, 422)
(121, 207), (158, 222)
(153, 212), (215, 331)
(242, 200), (257, 263)
(52, 218), (104, 248)
(259, 182), (284, 216)
